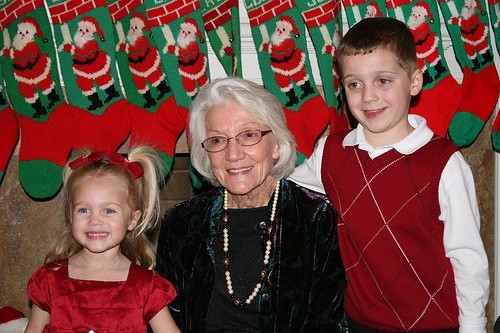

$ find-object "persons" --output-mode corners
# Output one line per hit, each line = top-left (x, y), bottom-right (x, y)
(24, 143), (180, 333)
(284, 18), (489, 333)
(152, 76), (349, 333)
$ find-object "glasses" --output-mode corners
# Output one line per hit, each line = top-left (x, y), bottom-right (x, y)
(201, 130), (271, 152)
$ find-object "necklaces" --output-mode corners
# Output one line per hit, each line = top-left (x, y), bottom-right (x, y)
(223, 179), (280, 306)
